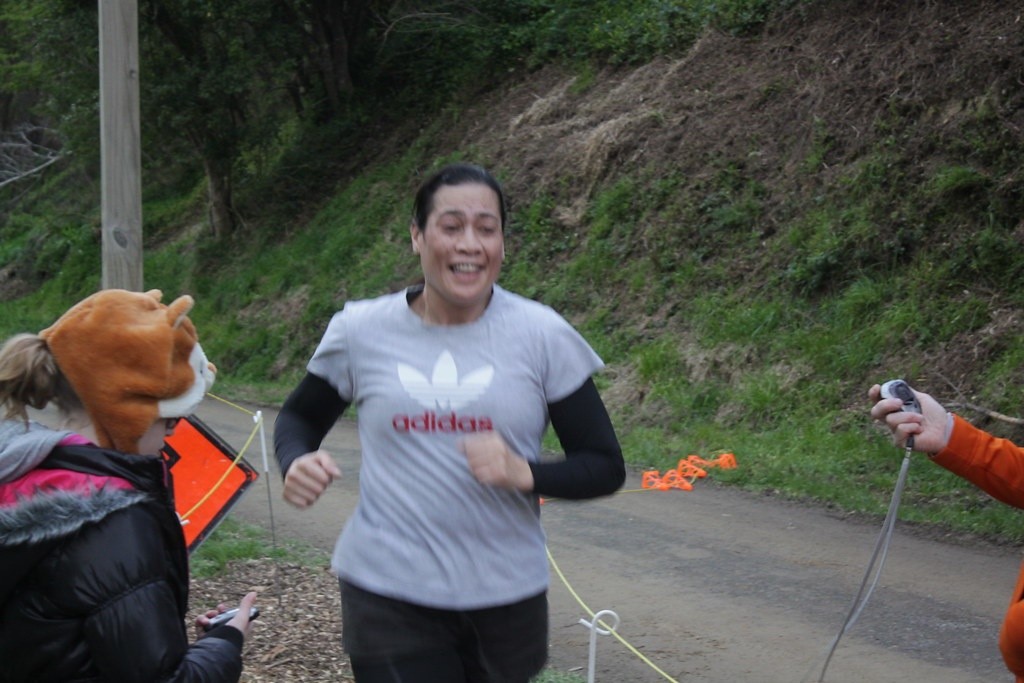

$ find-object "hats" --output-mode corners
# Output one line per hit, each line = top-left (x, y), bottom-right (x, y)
(38, 288), (218, 456)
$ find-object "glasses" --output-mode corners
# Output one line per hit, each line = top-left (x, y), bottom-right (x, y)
(159, 417), (181, 429)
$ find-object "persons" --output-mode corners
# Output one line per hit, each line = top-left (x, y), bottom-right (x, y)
(273, 165), (627, 683)
(0, 287), (257, 683)
(868, 383), (1024, 683)
